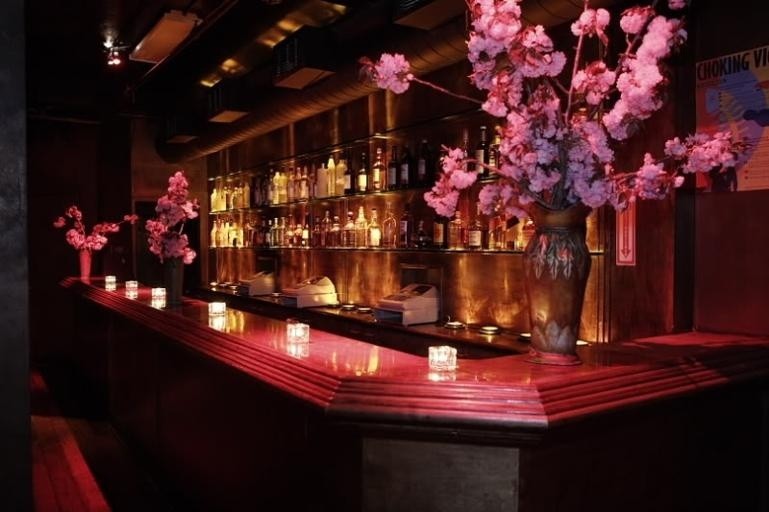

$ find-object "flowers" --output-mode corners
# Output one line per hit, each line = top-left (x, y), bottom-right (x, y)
(359, 0), (757, 219)
(145, 172), (200, 264)
(53, 205), (137, 251)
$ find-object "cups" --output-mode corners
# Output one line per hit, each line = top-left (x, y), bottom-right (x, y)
(207, 300), (227, 316)
(125, 289), (138, 301)
(286, 323), (310, 344)
(105, 282), (117, 291)
(126, 281), (138, 289)
(106, 275), (116, 282)
(283, 344), (311, 359)
(425, 374), (458, 383)
(208, 316), (229, 334)
(427, 344), (456, 372)
(151, 298), (166, 309)
(151, 287), (166, 299)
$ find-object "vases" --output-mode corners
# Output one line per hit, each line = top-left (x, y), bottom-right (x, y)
(165, 257), (184, 305)
(522, 202), (592, 366)
(80, 251), (91, 284)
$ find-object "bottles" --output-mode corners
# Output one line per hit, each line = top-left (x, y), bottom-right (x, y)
(227, 307), (249, 332)
(366, 207), (380, 247)
(265, 220), (272, 246)
(329, 217), (342, 246)
(211, 219), (216, 247)
(468, 203), (487, 249)
(221, 218), (226, 248)
(505, 205), (521, 250)
(380, 202), (397, 248)
(209, 123), (506, 212)
(411, 215), (432, 247)
(521, 209), (541, 250)
(303, 215), (312, 247)
(313, 216), (320, 247)
(398, 203), (410, 246)
(215, 219), (221, 247)
(488, 207), (504, 250)
(342, 213), (356, 246)
(320, 209), (331, 246)
(272, 217), (279, 247)
(355, 206), (366, 248)
(225, 218), (232, 247)
(449, 210), (468, 249)
(294, 223), (302, 247)
(286, 214), (294, 246)
(280, 216), (285, 247)
(433, 207), (448, 246)
(311, 330), (400, 380)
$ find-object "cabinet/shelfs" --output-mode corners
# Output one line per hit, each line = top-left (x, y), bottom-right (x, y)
(196, 109), (610, 361)
